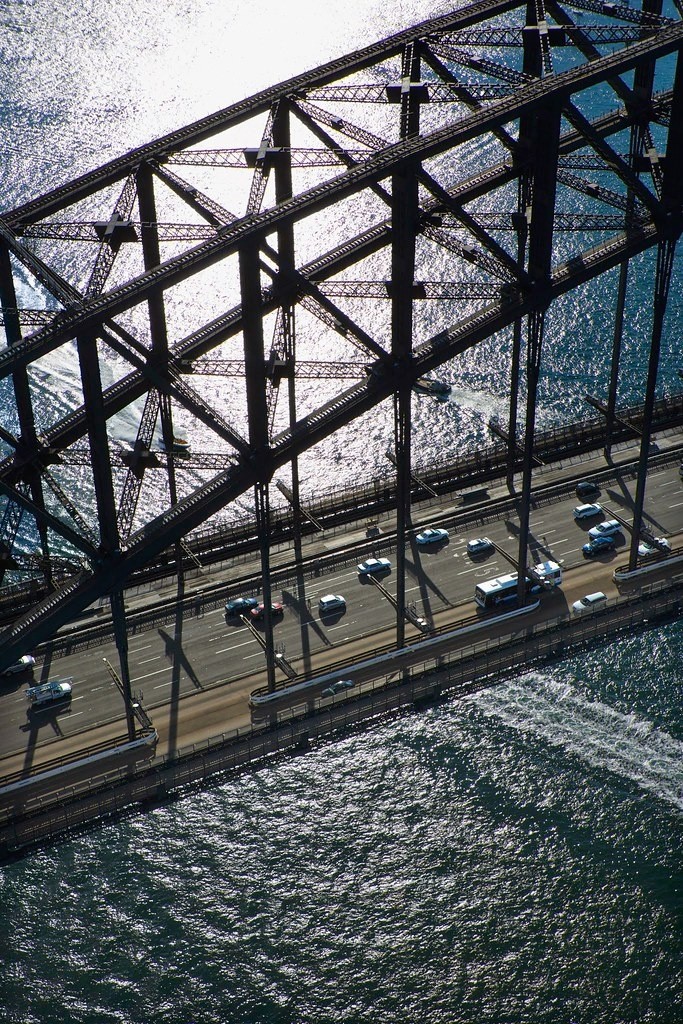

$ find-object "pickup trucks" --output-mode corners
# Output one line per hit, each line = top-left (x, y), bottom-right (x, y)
(25, 682), (72, 706)
(587, 519), (623, 541)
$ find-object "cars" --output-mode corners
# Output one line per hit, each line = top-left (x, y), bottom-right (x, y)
(250, 602), (283, 620)
(356, 557), (391, 574)
(467, 538), (493, 554)
(582, 537), (615, 557)
(415, 527), (450, 545)
(0, 653), (36, 677)
(572, 503), (603, 519)
(576, 480), (597, 496)
(224, 596), (258, 616)
(572, 592), (608, 613)
(638, 536), (673, 558)
(318, 594), (347, 614)
(320, 679), (354, 699)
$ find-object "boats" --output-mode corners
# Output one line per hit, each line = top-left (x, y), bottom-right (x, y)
(415, 376), (452, 395)
(156, 433), (192, 452)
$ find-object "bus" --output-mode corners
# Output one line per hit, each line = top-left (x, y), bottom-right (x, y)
(474, 561), (562, 609)
